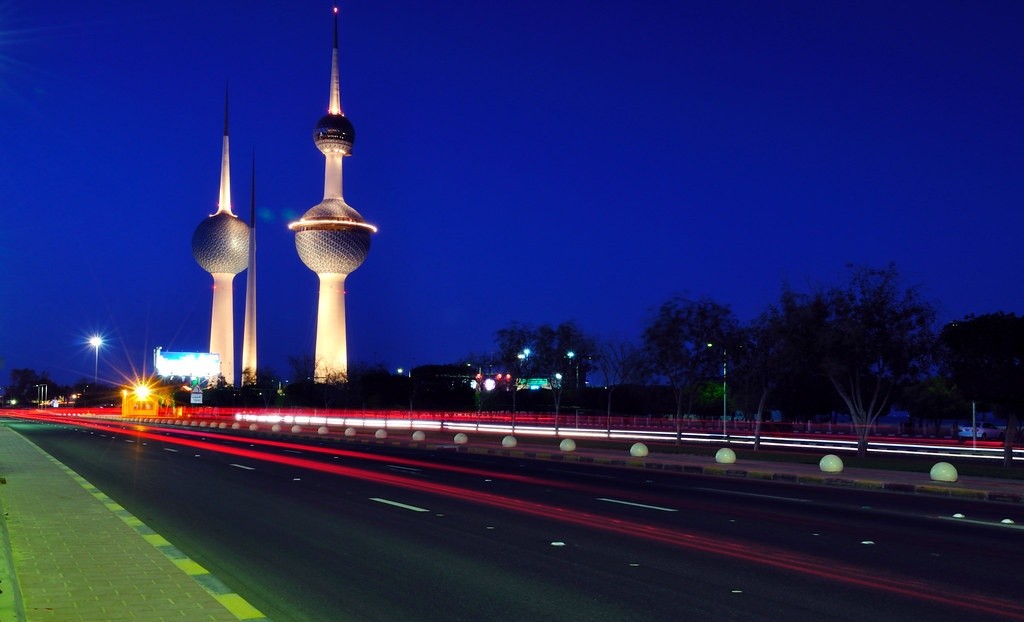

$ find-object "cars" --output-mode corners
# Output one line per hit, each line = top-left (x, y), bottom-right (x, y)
(959, 421), (1004, 442)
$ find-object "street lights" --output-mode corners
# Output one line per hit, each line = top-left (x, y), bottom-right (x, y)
(33, 383), (49, 410)
(705, 340), (743, 439)
(948, 311), (982, 450)
(464, 359), (494, 415)
(566, 351), (580, 415)
(89, 333), (101, 387)
(397, 367), (415, 416)
(308, 375), (331, 411)
